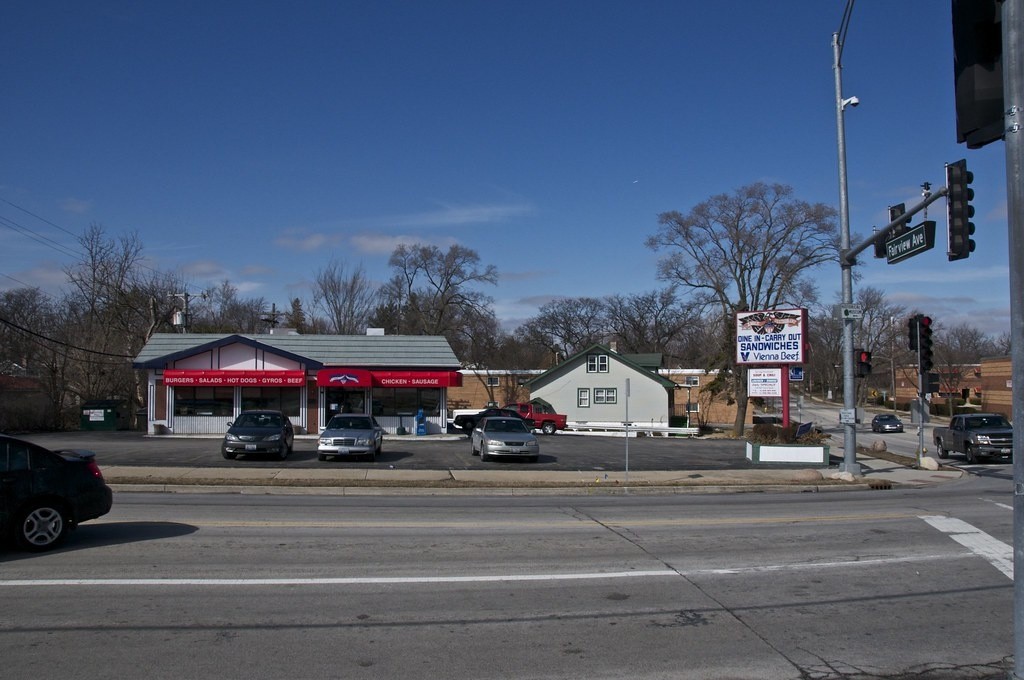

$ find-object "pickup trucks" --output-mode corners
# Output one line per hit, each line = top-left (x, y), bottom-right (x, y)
(933, 414), (1013, 464)
(501, 403), (567, 435)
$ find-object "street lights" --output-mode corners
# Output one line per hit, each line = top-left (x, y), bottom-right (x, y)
(871, 356), (896, 412)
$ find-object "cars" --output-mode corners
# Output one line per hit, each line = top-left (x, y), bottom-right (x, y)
(871, 414), (903, 433)
(470, 417), (540, 462)
(0, 435), (113, 553)
(453, 408), (535, 430)
(221, 410), (294, 461)
(317, 413), (382, 462)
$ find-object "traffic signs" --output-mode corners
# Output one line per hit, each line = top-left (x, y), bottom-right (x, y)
(885, 221), (936, 264)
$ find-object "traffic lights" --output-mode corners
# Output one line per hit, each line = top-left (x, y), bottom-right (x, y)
(888, 202), (912, 237)
(908, 313), (933, 370)
(944, 158), (976, 262)
(854, 347), (872, 378)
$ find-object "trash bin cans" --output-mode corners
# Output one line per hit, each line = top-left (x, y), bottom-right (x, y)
(81, 399), (129, 431)
(136, 407), (148, 431)
(670, 416), (687, 436)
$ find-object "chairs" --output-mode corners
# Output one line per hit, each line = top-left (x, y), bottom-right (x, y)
(243, 416), (280, 426)
